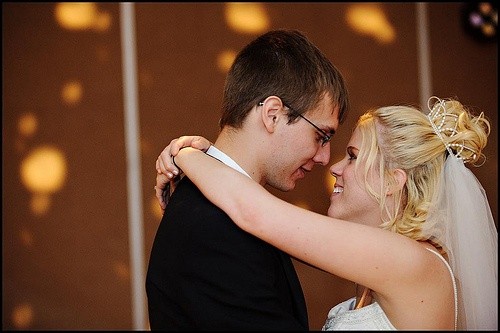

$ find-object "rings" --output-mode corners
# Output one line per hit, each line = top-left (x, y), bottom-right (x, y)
(155, 186), (157, 190)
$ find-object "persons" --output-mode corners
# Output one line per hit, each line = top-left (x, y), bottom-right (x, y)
(154, 95), (498, 332)
(145, 30), (349, 332)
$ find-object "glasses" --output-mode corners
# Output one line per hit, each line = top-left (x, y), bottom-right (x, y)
(259, 101), (332, 148)
